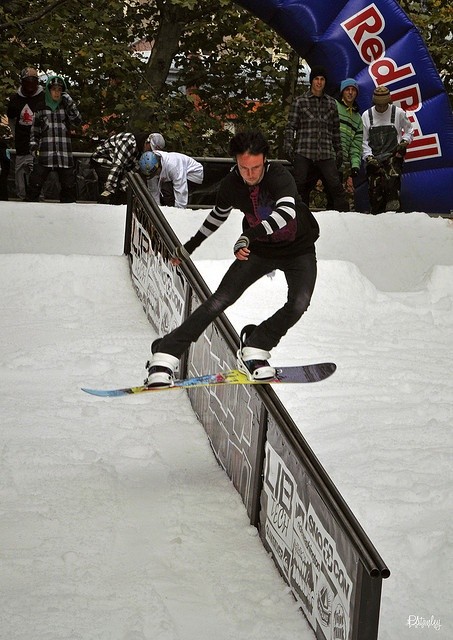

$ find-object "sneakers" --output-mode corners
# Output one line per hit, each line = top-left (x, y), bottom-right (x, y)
(243, 344), (277, 379)
(147, 352), (172, 385)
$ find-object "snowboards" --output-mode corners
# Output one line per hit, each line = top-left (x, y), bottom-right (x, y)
(81, 361), (338, 396)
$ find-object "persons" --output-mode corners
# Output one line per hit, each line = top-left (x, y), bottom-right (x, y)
(88, 132), (166, 204)
(326, 77), (363, 209)
(361, 86), (414, 215)
(138, 150), (210, 208)
(23, 75), (83, 203)
(283, 66), (350, 211)
(6, 66), (47, 201)
(144, 130), (320, 388)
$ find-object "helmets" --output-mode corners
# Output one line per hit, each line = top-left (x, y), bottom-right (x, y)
(139, 152), (160, 180)
(147, 132), (166, 150)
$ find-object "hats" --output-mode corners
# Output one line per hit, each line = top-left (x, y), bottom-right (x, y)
(49, 78), (65, 87)
(372, 86), (391, 104)
(341, 78), (359, 93)
(21, 67), (38, 78)
(309, 68), (327, 82)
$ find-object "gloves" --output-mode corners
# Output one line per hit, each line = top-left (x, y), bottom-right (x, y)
(234, 237), (250, 254)
(396, 140), (409, 159)
(336, 151), (343, 168)
(29, 142), (39, 159)
(98, 190), (112, 204)
(366, 156), (380, 170)
(60, 91), (74, 106)
(172, 246), (190, 263)
